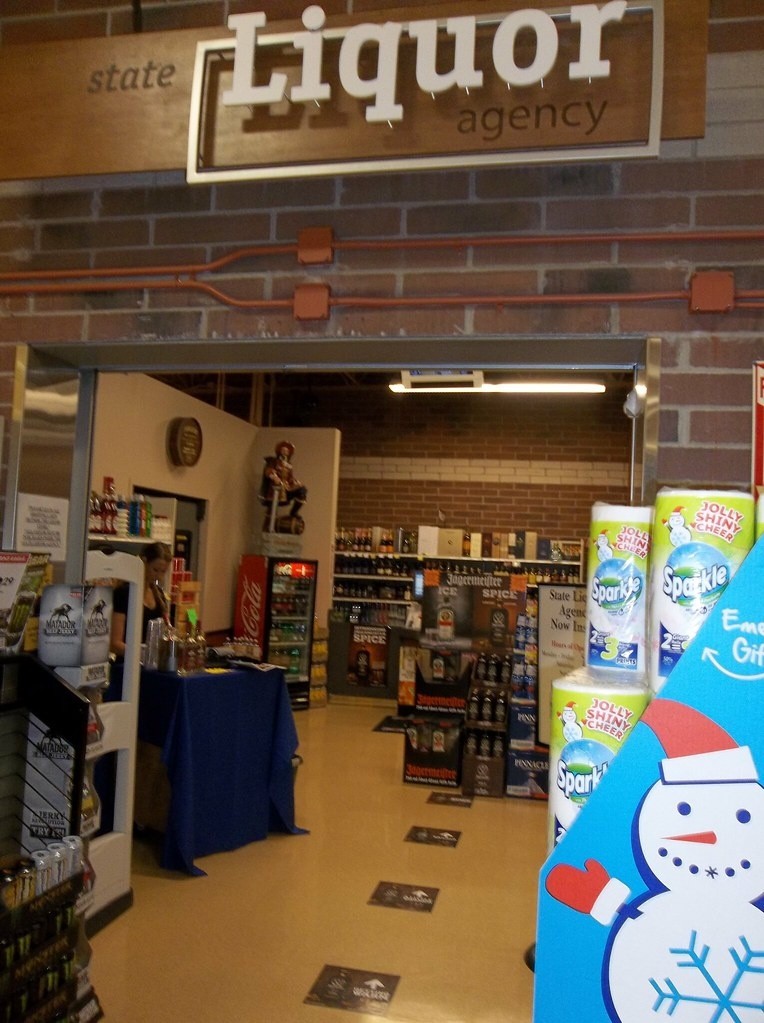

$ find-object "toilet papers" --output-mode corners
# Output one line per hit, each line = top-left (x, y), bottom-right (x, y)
(546, 488), (764, 858)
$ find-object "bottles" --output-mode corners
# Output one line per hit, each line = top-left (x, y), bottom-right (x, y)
(332, 527), (581, 628)
(176, 629), (206, 675)
(355, 642), (371, 677)
(268, 597), (308, 672)
(489, 598), (509, 647)
(465, 651), (510, 756)
(431, 650), (446, 683)
(88, 489), (172, 544)
(436, 598), (456, 640)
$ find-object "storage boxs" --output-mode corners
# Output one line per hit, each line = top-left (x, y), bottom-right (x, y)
(38, 583), (114, 667)
(413, 653), (474, 714)
(404, 712), (466, 790)
(417, 525), (550, 562)
(463, 755), (504, 798)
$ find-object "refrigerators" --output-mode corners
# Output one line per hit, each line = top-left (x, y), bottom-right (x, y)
(233, 554), (319, 710)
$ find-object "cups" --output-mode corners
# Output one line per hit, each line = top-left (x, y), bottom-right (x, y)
(159, 640), (177, 672)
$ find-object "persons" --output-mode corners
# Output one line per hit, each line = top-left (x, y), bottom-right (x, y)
(258, 440), (307, 533)
(110, 541), (173, 663)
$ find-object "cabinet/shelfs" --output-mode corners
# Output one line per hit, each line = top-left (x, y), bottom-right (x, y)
(86, 496), (176, 626)
(328, 541), (584, 798)
(95, 655), (312, 878)
(22, 552), (144, 942)
(0, 653), (105, 1023)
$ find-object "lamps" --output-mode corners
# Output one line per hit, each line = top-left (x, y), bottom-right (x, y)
(387, 375), (607, 392)
